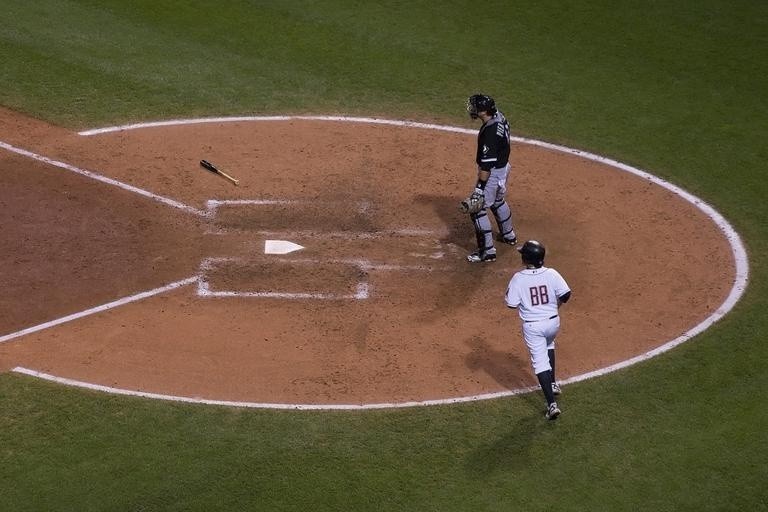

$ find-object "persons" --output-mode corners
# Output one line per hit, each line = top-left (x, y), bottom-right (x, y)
(502, 238), (574, 421)
(462, 91), (518, 265)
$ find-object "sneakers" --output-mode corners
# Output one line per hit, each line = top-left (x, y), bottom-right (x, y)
(467, 252), (496, 262)
(497, 233), (518, 246)
(545, 402), (560, 420)
(551, 381), (562, 394)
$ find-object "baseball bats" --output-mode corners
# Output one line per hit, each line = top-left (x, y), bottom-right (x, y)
(200, 160), (239, 186)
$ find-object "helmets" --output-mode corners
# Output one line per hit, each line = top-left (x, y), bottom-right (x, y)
(464, 94), (497, 122)
(517, 240), (545, 265)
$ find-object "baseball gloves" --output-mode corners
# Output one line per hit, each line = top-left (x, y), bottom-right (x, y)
(457, 192), (485, 213)
